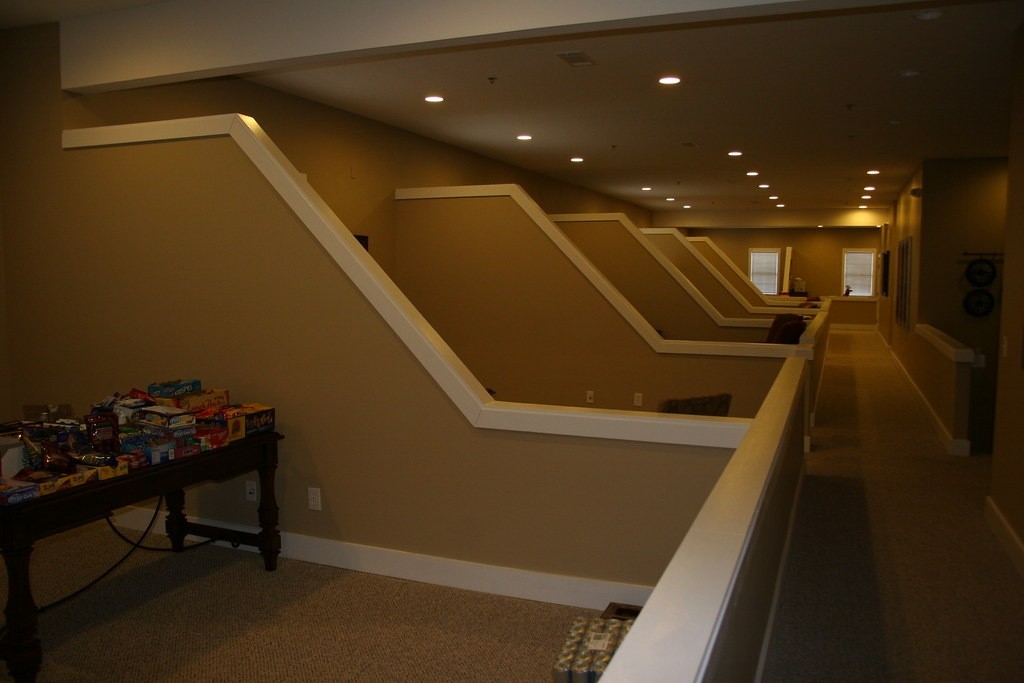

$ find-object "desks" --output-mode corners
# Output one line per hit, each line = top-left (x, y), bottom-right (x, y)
(0, 431), (286, 683)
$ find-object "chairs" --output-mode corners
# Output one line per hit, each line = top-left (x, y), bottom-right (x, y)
(766, 312), (807, 344)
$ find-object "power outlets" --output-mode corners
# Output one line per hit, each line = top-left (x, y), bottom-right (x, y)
(245, 480), (257, 502)
(309, 487), (322, 510)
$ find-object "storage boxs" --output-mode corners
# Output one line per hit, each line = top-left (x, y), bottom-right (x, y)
(124, 433), (175, 466)
(0, 479), (39, 508)
(115, 449), (147, 472)
(147, 379), (201, 398)
(196, 405), (276, 435)
(193, 424), (228, 451)
(156, 388), (231, 411)
(93, 457), (129, 481)
(196, 416), (246, 441)
(173, 438), (201, 457)
(40, 465), (100, 496)
(141, 406), (195, 428)
